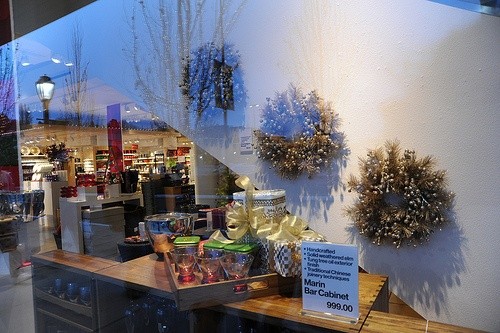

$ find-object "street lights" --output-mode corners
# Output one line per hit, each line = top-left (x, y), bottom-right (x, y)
(35, 75), (57, 124)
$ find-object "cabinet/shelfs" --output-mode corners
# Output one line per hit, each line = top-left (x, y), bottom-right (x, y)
(20, 145), (387, 333)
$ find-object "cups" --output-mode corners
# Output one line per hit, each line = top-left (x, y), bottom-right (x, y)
(54, 270), (66, 299)
(171, 248), (197, 283)
(66, 283), (79, 303)
(79, 286), (90, 306)
(219, 253), (254, 292)
(192, 250), (223, 284)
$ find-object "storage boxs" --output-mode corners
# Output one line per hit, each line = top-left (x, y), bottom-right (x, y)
(266, 230), (328, 278)
(233, 189), (287, 219)
(163, 252), (280, 312)
